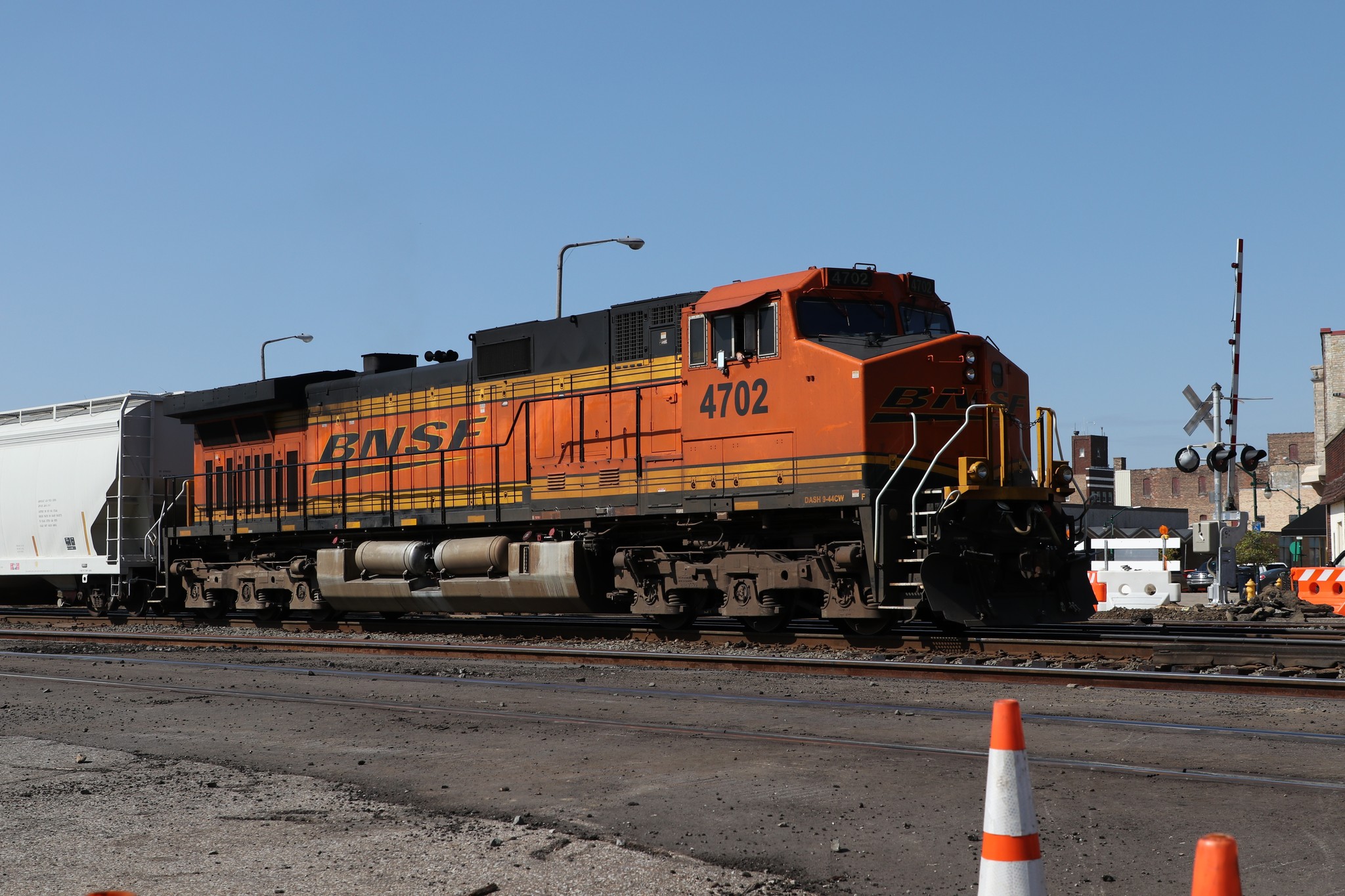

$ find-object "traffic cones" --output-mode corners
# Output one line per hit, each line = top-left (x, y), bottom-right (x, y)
(1190, 833), (1242, 896)
(976, 698), (1049, 896)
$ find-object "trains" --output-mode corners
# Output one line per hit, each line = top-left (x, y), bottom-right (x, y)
(0, 264), (1099, 652)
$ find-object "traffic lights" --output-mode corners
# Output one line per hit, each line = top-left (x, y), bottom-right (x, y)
(1211, 445), (1237, 472)
(1240, 445), (1267, 472)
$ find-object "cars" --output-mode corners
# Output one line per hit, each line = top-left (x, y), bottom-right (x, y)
(1185, 561), (1299, 593)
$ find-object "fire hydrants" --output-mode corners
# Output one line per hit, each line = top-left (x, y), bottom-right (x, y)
(1242, 578), (1257, 601)
(1274, 577), (1282, 590)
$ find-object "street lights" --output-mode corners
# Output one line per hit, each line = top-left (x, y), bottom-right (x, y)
(1102, 506), (1141, 560)
(261, 333), (314, 381)
(1235, 463), (1273, 595)
(554, 236), (645, 318)
(1261, 456), (1310, 568)
